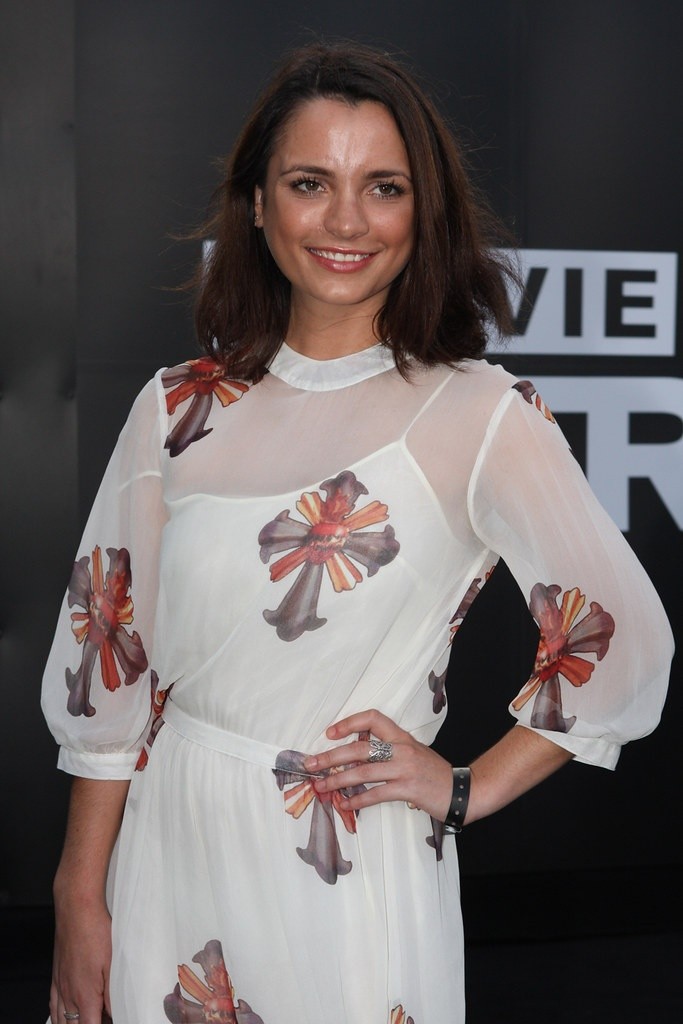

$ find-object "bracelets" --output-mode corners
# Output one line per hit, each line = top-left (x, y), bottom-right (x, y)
(443, 767), (471, 833)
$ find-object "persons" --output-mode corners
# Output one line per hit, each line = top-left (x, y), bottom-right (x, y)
(36, 46), (675, 1024)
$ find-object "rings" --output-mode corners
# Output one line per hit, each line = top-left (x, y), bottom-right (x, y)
(63, 1010), (79, 1020)
(367, 739), (393, 764)
